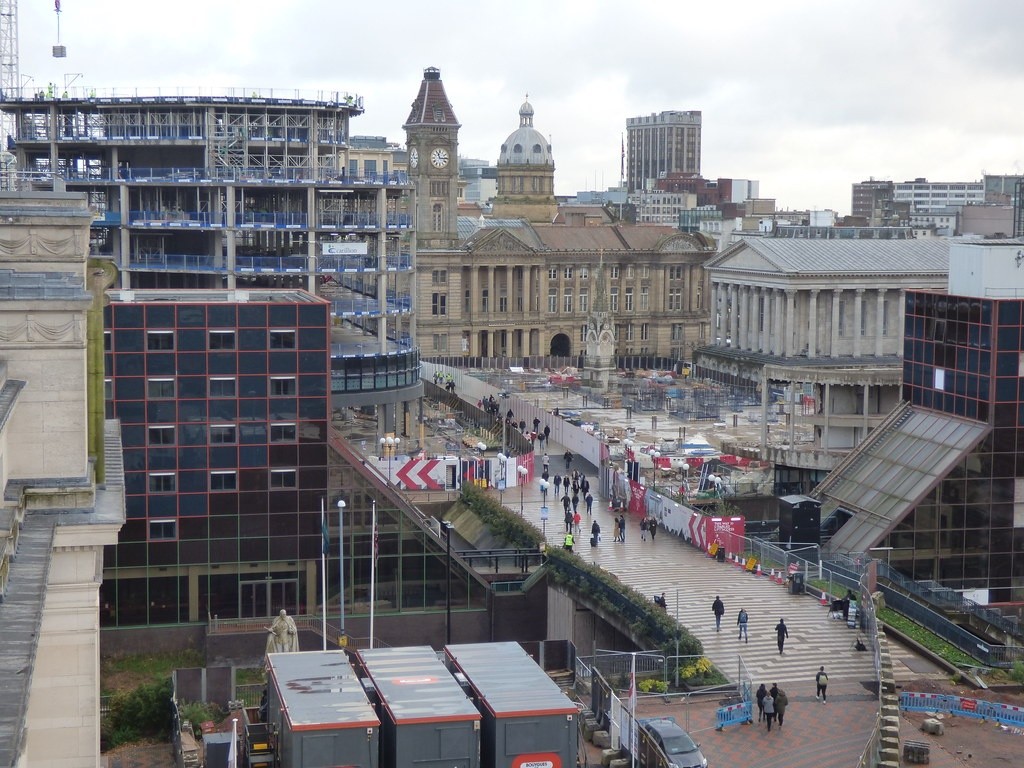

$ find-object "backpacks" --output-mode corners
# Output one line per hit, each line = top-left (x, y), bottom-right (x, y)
(740, 612), (747, 623)
(818, 672), (827, 686)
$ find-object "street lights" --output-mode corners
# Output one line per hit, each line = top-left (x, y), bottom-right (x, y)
(477, 441), (487, 457)
(708, 475), (721, 510)
(678, 461), (689, 505)
(540, 479), (550, 536)
(338, 500), (346, 649)
(517, 465), (528, 519)
(650, 450), (660, 491)
(442, 521), (455, 645)
(497, 453), (507, 506)
(379, 437), (401, 481)
(624, 439), (634, 472)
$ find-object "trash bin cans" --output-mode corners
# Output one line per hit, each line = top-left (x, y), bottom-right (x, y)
(717, 547), (725, 562)
(786, 572), (803, 595)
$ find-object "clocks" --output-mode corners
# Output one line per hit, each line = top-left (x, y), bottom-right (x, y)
(409, 147), (418, 168)
(430, 148), (449, 169)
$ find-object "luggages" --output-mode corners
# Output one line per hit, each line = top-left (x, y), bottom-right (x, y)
(590, 533), (596, 547)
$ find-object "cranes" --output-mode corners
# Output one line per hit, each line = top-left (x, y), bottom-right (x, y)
(0, 0), (66, 103)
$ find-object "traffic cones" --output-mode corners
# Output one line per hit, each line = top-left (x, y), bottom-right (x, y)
(821, 588), (827, 606)
(769, 568), (776, 581)
(727, 551), (746, 569)
(775, 571), (785, 585)
(608, 500), (613, 511)
(756, 562), (762, 577)
(619, 502), (624, 512)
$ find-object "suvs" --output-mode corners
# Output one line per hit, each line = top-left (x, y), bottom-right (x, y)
(644, 720), (708, 768)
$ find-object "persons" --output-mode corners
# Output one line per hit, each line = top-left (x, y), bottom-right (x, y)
(591, 520), (599, 540)
(90, 90), (94, 96)
(40, 82), (54, 97)
(343, 96), (352, 102)
(268, 610), (295, 652)
(433, 371), (455, 393)
(539, 450), (593, 533)
(564, 532), (575, 551)
(844, 589), (856, 600)
(712, 596), (724, 631)
(613, 515), (625, 542)
(252, 92), (257, 97)
(775, 619), (788, 654)
(816, 666), (828, 704)
(660, 593), (667, 610)
(756, 684), (788, 730)
(639, 517), (656, 541)
(497, 447), (503, 453)
(62, 91), (68, 97)
(478, 395), (550, 450)
(737, 609), (748, 643)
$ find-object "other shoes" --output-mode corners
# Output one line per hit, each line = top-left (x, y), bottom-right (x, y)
(816, 695), (820, 699)
(823, 700), (826, 704)
